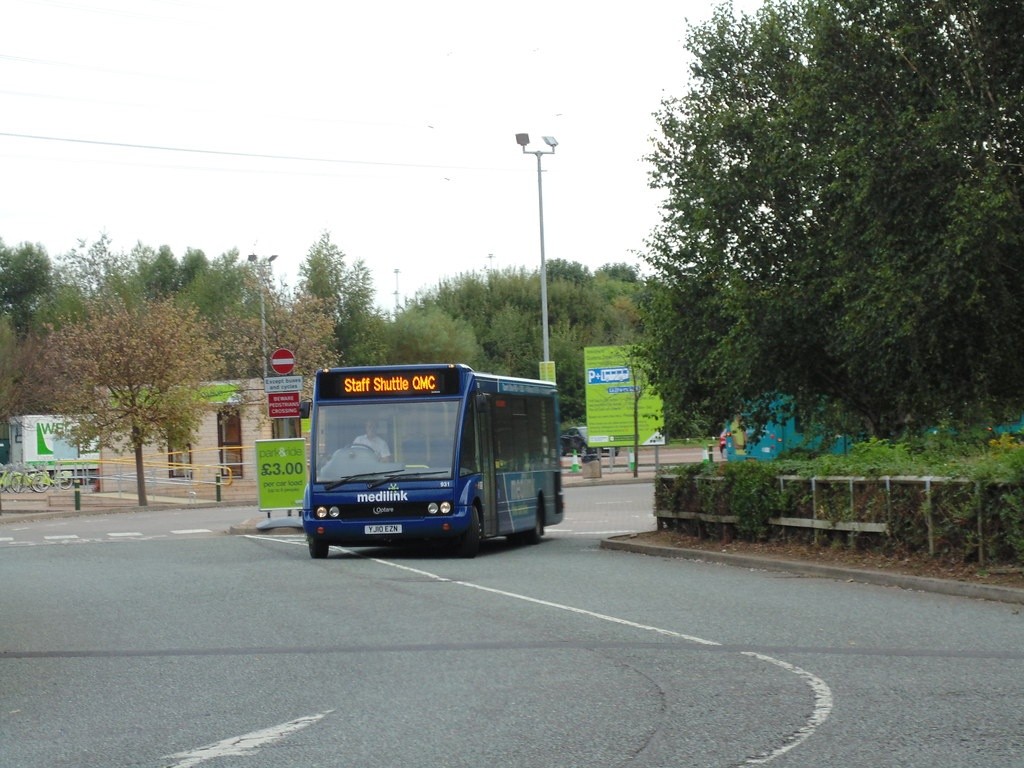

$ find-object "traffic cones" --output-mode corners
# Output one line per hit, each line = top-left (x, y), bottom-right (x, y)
(626, 448), (635, 472)
(568, 448), (581, 474)
(700, 440), (708, 465)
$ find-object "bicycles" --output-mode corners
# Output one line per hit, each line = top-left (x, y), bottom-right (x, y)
(0, 458), (73, 494)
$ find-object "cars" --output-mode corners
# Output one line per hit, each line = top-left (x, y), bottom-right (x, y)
(560, 426), (620, 459)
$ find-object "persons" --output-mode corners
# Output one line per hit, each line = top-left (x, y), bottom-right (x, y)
(351, 419), (391, 464)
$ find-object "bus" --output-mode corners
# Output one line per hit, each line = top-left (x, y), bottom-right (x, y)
(299, 363), (564, 559)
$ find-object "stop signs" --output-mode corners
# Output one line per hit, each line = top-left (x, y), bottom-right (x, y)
(270, 349), (296, 375)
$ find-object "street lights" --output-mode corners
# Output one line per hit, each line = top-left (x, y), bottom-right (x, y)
(248, 254), (278, 437)
(516, 133), (559, 381)
